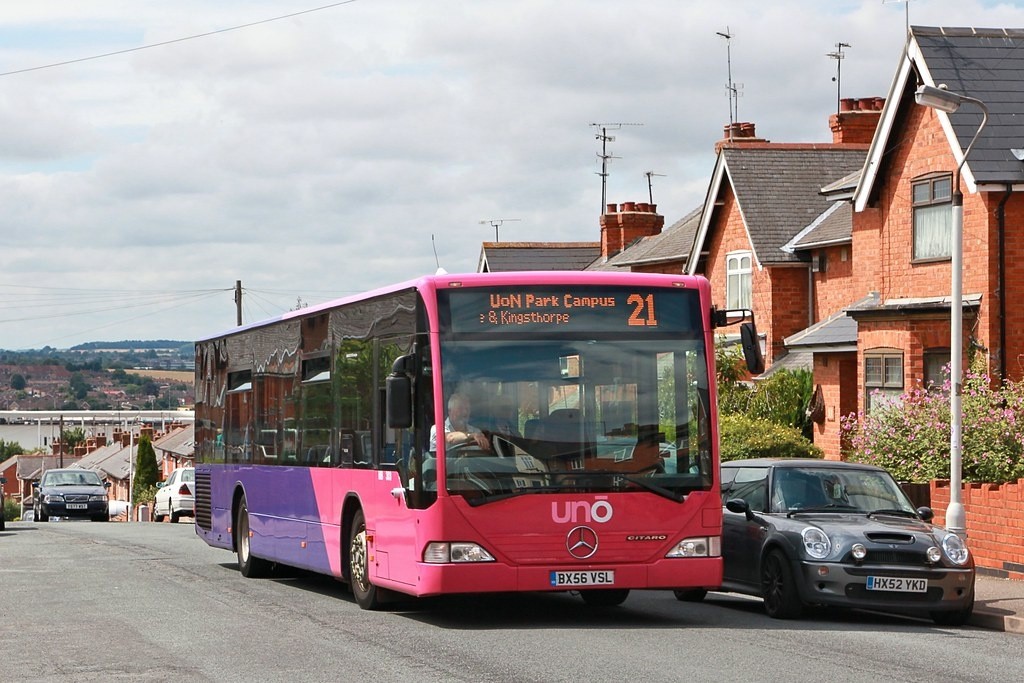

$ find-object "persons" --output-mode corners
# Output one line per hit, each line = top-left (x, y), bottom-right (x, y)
(429, 392), (489, 451)
(479, 395), (526, 451)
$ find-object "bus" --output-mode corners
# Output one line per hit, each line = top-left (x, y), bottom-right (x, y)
(193, 271), (766, 611)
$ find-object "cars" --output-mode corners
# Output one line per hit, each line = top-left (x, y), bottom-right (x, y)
(153, 468), (194, 523)
(673, 459), (975, 627)
(32, 468), (112, 522)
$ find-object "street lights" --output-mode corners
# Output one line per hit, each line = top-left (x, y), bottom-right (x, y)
(916, 83), (990, 550)
